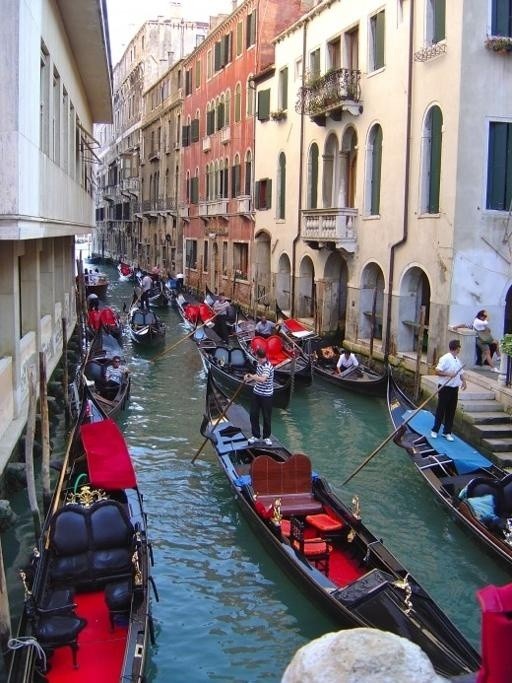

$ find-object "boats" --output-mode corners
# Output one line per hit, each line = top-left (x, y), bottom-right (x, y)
(382, 357), (512, 573)
(189, 356), (483, 679)
(2, 358), (165, 682)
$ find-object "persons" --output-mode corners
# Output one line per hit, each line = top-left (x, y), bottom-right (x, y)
(175, 272), (184, 293)
(90, 270), (94, 284)
(243, 349), (275, 446)
(104, 356), (130, 387)
(453, 315), (497, 367)
(93, 268), (99, 285)
(336, 350), (358, 374)
(474, 310), (500, 372)
(431, 339), (467, 442)
(85, 269), (89, 284)
(254, 315), (279, 338)
(213, 292), (231, 345)
(151, 264), (160, 287)
(138, 270), (152, 311)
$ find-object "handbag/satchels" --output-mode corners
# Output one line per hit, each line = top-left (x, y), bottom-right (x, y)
(478, 328), (493, 344)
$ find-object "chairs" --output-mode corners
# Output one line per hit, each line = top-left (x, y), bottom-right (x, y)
(304, 339), (340, 367)
(88, 308), (114, 330)
(290, 514), (328, 578)
(186, 303), (209, 321)
(18, 568), (84, 675)
(133, 312), (157, 329)
(214, 347), (250, 374)
(50, 500), (134, 591)
(86, 360), (113, 391)
(468, 474), (512, 531)
(251, 336), (285, 365)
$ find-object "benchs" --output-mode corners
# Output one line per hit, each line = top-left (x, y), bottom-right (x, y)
(252, 454), (323, 517)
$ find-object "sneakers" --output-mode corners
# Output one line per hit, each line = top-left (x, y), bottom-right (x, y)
(248, 437), (259, 444)
(431, 431), (437, 438)
(491, 367), (498, 373)
(442, 434), (453, 441)
(262, 438), (272, 445)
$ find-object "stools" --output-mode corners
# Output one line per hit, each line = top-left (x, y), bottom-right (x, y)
(306, 514), (342, 538)
(43, 590), (72, 615)
(279, 519), (300, 540)
(104, 581), (141, 632)
